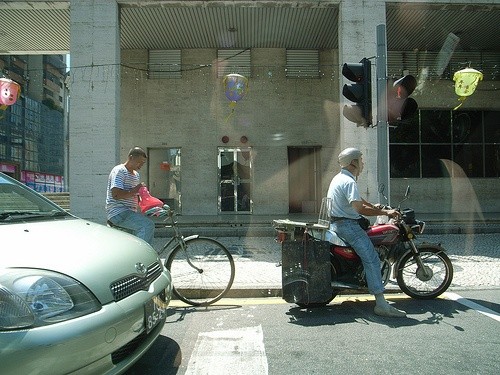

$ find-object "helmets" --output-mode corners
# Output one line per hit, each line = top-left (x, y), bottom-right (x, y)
(338, 148), (363, 168)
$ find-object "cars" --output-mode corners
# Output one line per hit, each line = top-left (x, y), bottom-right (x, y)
(0, 171), (172, 375)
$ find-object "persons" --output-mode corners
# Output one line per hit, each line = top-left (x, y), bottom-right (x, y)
(326, 148), (407, 319)
(106, 146), (155, 248)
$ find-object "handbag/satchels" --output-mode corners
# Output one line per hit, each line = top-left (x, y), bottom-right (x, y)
(137, 185), (164, 214)
(357, 218), (370, 230)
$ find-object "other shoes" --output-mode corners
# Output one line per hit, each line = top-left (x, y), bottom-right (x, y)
(374, 304), (406, 317)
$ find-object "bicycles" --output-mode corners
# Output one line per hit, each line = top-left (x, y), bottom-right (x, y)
(105, 206), (235, 306)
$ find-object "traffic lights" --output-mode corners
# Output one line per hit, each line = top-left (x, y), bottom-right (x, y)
(340, 57), (373, 128)
(385, 76), (418, 125)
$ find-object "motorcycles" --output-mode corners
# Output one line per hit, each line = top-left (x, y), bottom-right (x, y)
(272, 183), (454, 307)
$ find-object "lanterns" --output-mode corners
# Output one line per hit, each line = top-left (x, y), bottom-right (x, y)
(453, 66), (484, 111)
(222, 73), (249, 123)
(0, 78), (22, 110)
